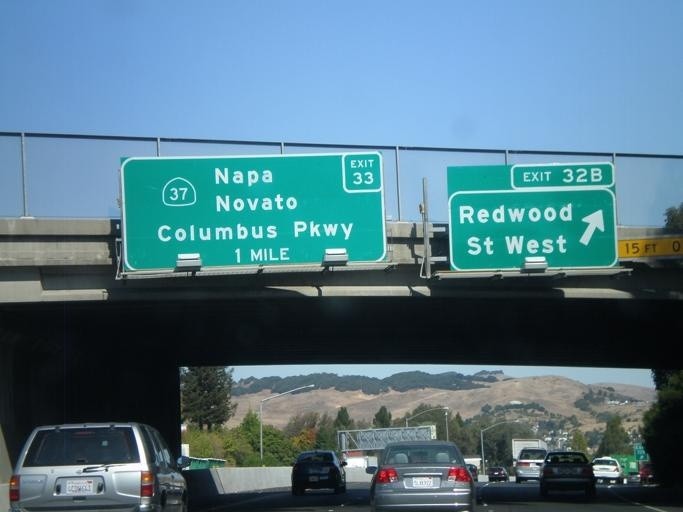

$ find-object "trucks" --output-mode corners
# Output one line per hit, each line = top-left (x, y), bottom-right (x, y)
(511, 438), (548, 476)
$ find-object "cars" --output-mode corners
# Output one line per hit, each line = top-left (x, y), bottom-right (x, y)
(8, 422), (190, 512)
(484, 447), (641, 497)
(366, 438), (478, 511)
(290, 450), (348, 496)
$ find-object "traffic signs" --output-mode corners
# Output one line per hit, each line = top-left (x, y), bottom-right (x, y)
(445, 159), (621, 272)
(634, 443), (649, 461)
(116, 148), (390, 272)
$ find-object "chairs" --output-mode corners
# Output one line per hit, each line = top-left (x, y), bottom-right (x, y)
(521, 452), (583, 464)
(392, 453), (407, 464)
(436, 453), (449, 464)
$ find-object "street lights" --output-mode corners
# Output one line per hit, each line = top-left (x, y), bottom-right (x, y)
(479, 420), (518, 475)
(259, 383), (316, 464)
